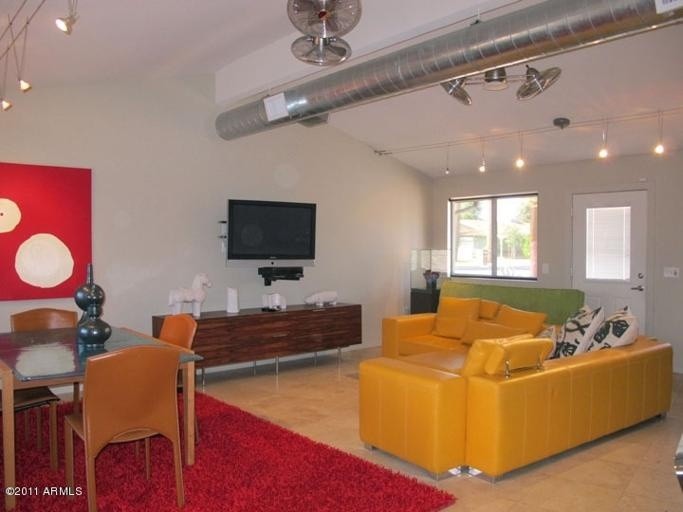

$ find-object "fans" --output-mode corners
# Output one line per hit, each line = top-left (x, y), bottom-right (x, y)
(283, 0), (362, 72)
(440, 62), (562, 107)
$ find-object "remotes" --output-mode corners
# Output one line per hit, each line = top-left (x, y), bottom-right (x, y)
(261, 307), (279, 312)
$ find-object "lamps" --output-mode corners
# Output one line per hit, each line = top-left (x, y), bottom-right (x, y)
(421, 249), (449, 290)
(0, 0), (82, 113)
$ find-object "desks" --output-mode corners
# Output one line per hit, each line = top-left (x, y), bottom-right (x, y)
(0, 324), (205, 512)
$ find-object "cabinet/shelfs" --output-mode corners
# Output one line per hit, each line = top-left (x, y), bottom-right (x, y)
(151, 301), (362, 388)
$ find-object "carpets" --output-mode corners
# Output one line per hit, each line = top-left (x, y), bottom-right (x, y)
(0, 387), (457, 512)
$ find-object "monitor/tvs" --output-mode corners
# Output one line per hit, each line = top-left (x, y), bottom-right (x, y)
(224, 198), (318, 268)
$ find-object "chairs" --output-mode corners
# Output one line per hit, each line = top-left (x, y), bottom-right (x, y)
(8, 308), (197, 512)
